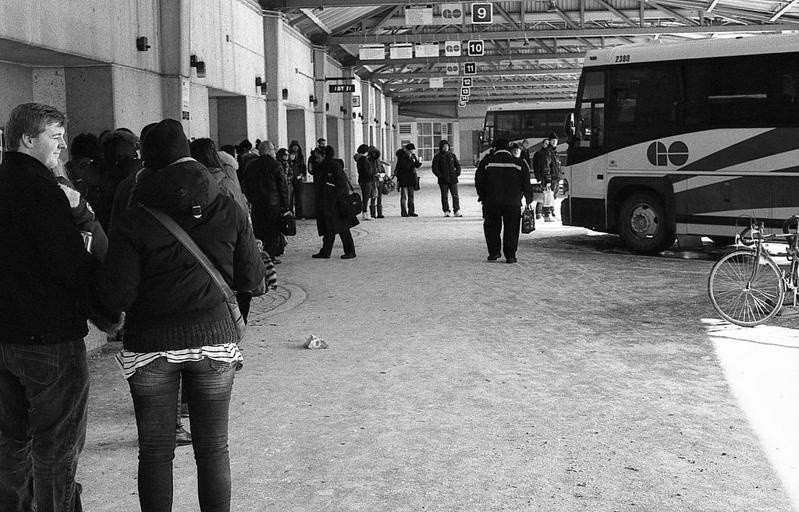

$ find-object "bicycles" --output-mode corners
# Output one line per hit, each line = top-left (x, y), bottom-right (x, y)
(705, 209), (798, 329)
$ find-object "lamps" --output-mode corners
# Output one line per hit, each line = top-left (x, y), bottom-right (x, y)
(256, 77), (270, 96)
(191, 54), (206, 79)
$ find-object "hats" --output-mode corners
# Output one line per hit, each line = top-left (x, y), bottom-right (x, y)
(549, 131), (558, 141)
(142, 118), (191, 168)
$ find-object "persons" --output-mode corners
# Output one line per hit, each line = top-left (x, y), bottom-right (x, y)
(429, 139), (465, 219)
(0, 100), (109, 511)
(58, 126), (307, 451)
(95, 114), (268, 512)
(472, 130), (564, 264)
(306, 133), (425, 260)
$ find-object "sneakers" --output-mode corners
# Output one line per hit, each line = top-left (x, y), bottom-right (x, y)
(362, 205), (463, 220)
(506, 258), (517, 263)
(181, 404), (190, 417)
(536, 211), (556, 222)
(176, 429), (192, 445)
(487, 250), (501, 260)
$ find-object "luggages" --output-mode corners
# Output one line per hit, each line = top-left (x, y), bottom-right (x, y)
(294, 182), (317, 220)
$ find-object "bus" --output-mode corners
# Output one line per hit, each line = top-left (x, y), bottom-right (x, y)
(563, 34), (799, 251)
(473, 100), (603, 198)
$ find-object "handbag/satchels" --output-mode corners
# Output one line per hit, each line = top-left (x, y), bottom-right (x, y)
(381, 179), (395, 194)
(522, 209), (535, 233)
(281, 215), (296, 236)
(339, 192), (362, 217)
(414, 177), (420, 191)
(542, 190), (555, 207)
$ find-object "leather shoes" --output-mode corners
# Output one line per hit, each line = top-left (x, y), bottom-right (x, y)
(312, 252), (331, 258)
(341, 253), (356, 259)
